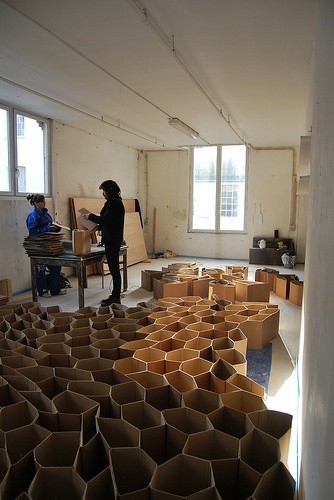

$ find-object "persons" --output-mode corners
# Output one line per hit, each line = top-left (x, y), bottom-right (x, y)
(26, 194), (68, 298)
(81, 179), (125, 307)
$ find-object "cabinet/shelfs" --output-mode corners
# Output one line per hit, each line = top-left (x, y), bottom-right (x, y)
(249, 236), (297, 266)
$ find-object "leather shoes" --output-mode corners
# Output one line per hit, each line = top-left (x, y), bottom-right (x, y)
(101, 298), (121, 306)
(102, 295), (113, 303)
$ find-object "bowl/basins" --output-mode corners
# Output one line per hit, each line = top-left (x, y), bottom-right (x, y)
(279, 246), (287, 249)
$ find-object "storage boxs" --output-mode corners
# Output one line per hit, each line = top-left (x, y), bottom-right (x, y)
(0, 221), (303, 500)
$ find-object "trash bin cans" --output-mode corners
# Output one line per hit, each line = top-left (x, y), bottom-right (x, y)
(281, 253), (297, 268)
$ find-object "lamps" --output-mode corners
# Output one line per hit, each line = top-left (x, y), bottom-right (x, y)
(168, 118), (199, 140)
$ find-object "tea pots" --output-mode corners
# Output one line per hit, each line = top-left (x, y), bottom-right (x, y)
(257, 239), (266, 248)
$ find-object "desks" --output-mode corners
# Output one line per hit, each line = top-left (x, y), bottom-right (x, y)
(30, 241), (129, 309)
